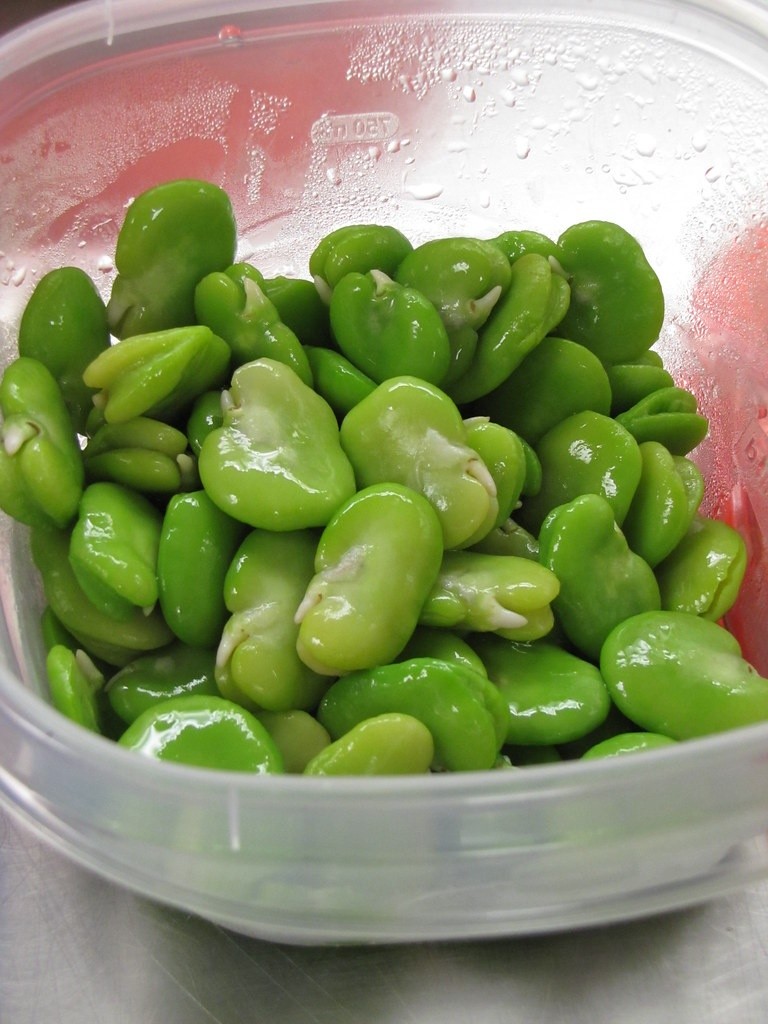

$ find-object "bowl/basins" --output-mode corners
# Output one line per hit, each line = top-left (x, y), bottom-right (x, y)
(0, 0), (768, 952)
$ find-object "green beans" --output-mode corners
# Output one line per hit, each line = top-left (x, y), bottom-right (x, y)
(0, 180), (768, 776)
